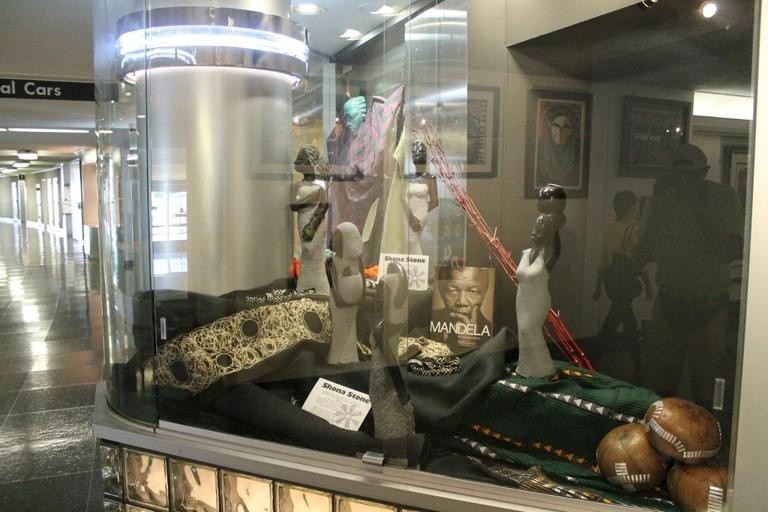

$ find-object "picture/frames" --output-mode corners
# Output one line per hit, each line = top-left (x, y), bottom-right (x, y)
(523, 88), (594, 200)
(618, 95), (694, 178)
(397, 79), (501, 180)
(724, 144), (749, 206)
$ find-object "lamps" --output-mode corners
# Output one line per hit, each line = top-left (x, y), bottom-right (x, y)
(697, 1), (719, 21)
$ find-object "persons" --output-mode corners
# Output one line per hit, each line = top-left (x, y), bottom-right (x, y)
(510, 182), (564, 374)
(289, 145), (331, 292)
(433, 266), (494, 358)
(398, 140), (432, 284)
(597, 144), (737, 405)
(321, 85), (405, 365)
